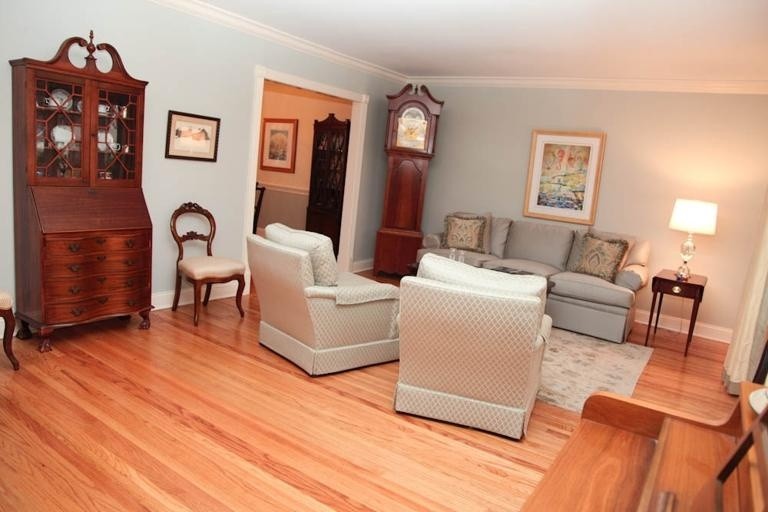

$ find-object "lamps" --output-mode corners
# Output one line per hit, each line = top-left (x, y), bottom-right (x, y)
(668, 198), (718, 281)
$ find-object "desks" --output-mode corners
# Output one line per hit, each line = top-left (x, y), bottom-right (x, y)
(645, 269), (709, 354)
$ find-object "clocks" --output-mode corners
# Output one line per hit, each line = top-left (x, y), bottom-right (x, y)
(372, 82), (445, 276)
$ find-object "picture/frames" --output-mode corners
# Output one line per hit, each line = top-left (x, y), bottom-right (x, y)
(523, 128), (606, 226)
(165, 110), (221, 162)
(260, 118), (299, 174)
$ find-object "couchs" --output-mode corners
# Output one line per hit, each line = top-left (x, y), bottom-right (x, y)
(415, 211), (651, 344)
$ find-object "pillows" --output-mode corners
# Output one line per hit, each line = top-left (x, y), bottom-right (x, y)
(443, 211), (627, 282)
(266, 222), (352, 288)
(416, 252), (546, 326)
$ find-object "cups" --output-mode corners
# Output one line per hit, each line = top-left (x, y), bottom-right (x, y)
(112, 143), (121, 152)
(99, 104), (110, 113)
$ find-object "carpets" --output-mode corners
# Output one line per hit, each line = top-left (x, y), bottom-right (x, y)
(537, 327), (656, 411)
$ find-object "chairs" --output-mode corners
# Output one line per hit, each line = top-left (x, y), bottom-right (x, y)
(168, 201), (246, 327)
(248, 233), (402, 377)
(1, 291), (21, 372)
(396, 275), (554, 443)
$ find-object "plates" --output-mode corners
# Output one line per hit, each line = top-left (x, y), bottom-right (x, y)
(48, 89), (73, 110)
(51, 124), (73, 145)
(98, 131), (112, 152)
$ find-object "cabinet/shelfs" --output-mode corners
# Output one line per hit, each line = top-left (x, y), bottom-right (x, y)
(9, 28), (155, 352)
